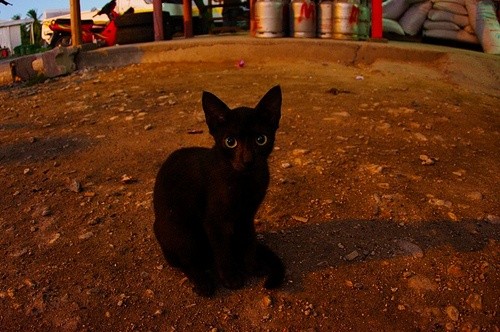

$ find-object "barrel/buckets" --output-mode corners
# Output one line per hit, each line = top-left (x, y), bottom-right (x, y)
(255, 0), (285, 38)
(332, 0), (361, 40)
(289, 0), (316, 38)
(317, 0), (333, 39)
(358, 0), (370, 41)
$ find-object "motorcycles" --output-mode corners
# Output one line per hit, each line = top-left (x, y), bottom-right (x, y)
(43, 0), (122, 52)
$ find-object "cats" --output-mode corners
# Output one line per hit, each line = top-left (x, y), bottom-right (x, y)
(152, 84), (286, 297)
